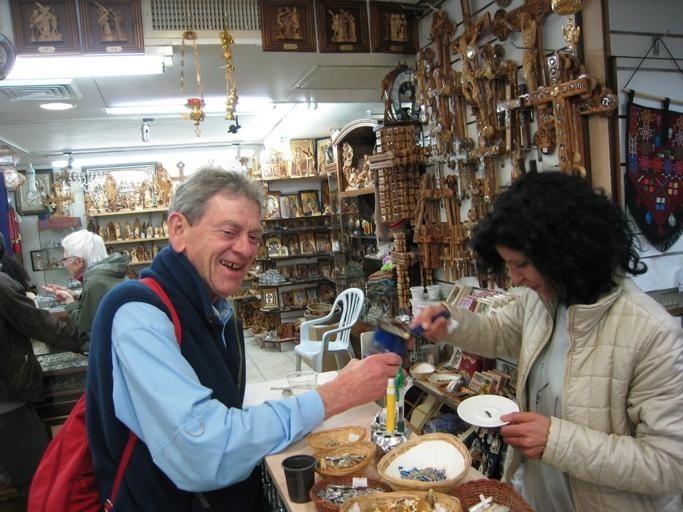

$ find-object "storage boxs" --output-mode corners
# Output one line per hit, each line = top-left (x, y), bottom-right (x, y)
(27, 348), (91, 421)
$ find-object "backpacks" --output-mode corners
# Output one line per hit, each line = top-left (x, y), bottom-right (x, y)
(24, 392), (108, 512)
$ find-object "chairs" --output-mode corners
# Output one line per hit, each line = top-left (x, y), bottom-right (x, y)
(291, 286), (365, 375)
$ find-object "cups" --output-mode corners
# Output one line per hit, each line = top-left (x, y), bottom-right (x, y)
(425, 285), (440, 299)
(281, 454), (316, 502)
(409, 286), (424, 301)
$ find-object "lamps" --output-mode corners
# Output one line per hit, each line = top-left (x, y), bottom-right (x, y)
(25, 162), (36, 194)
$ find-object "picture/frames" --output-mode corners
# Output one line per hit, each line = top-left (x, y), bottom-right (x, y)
(31, 249), (50, 271)
(368, 1), (419, 55)
(254, 136), (336, 310)
(15, 168), (54, 216)
(445, 283), (519, 396)
(80, 160), (160, 217)
(315, 0), (370, 54)
(81, 0), (143, 55)
(257, 1), (318, 52)
(8, 1), (81, 56)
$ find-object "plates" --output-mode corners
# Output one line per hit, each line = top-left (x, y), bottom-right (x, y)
(456, 394), (519, 427)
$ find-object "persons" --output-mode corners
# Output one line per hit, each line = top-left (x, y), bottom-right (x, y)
(42, 229), (129, 347)
(402, 172), (683, 511)
(0, 233), (36, 299)
(82, 169), (402, 511)
(0, 267), (80, 512)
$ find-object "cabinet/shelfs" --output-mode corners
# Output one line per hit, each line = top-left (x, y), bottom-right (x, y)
(254, 213), (337, 351)
(89, 205), (168, 280)
(36, 212), (83, 287)
(298, 322), (351, 373)
(333, 118), (396, 291)
(241, 369), (492, 512)
